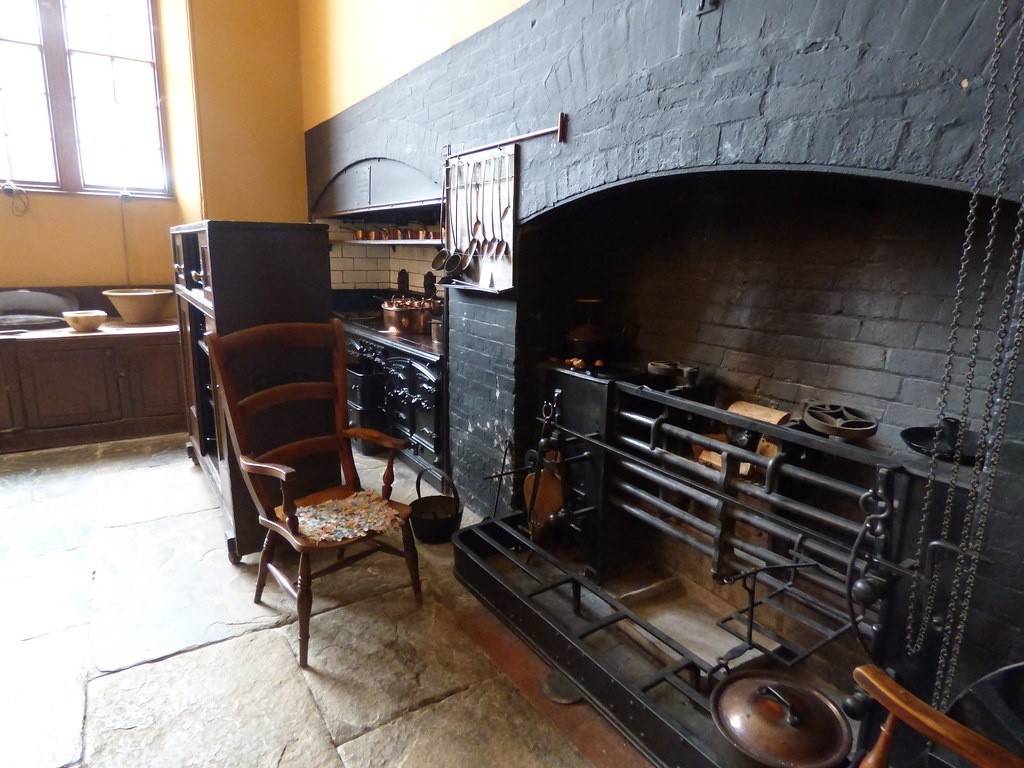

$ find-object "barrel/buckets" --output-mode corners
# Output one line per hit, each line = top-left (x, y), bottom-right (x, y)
(409, 467), (464, 544)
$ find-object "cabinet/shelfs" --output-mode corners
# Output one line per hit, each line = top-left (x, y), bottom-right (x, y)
(0, 334), (187, 457)
(167, 218), (331, 564)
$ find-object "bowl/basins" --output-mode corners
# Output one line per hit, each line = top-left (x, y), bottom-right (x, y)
(62, 310), (108, 332)
(101, 288), (174, 324)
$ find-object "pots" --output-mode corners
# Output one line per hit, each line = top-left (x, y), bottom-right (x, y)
(381, 294), (443, 334)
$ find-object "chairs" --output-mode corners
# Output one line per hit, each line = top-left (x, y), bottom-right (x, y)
(199, 315), (421, 671)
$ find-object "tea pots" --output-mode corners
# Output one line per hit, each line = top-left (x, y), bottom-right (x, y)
(532, 299), (639, 369)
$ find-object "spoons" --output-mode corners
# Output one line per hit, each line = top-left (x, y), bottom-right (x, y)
(430, 153), (506, 276)
